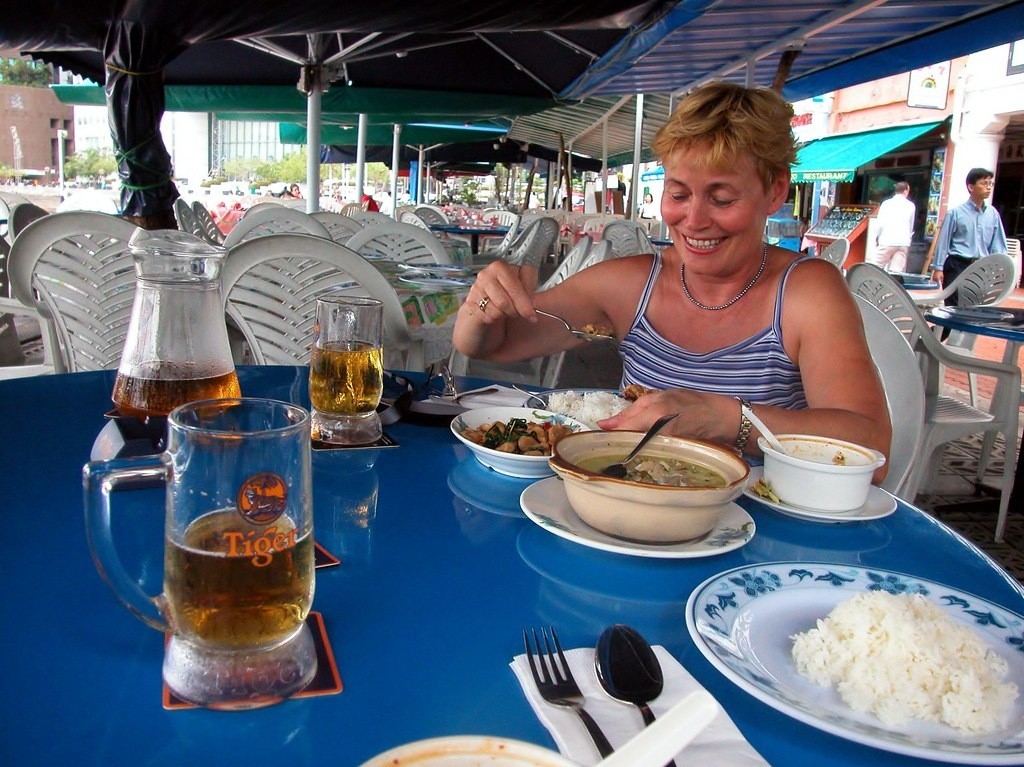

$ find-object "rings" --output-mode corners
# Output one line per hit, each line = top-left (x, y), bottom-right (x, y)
(479, 296), (489, 310)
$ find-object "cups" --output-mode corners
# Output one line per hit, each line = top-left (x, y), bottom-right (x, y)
(314, 451), (379, 577)
(81, 397), (319, 710)
(309, 295), (383, 445)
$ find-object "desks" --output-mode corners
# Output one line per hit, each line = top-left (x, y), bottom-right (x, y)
(428, 223), (524, 254)
(209, 207), (247, 236)
(312, 258), (477, 369)
(924, 305), (1024, 464)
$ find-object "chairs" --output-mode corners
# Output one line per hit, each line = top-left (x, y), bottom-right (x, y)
(0, 190), (1024, 539)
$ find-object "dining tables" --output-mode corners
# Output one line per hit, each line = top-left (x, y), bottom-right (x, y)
(0, 367), (1024, 767)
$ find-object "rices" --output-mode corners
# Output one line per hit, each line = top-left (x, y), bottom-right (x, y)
(788, 589), (1020, 733)
(546, 389), (635, 430)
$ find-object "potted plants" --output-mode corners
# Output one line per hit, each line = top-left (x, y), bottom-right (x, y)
(436, 176), (488, 206)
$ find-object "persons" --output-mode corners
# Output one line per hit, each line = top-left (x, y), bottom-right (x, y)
(282, 184), (301, 200)
(875, 182), (915, 275)
(639, 193), (658, 219)
(355, 188), (414, 215)
(453, 81), (891, 484)
(926, 168), (1006, 344)
(560, 196), (569, 210)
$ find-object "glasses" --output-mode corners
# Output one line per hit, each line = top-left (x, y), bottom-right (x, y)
(977, 182), (995, 187)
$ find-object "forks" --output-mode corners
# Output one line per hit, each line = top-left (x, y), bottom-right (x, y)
(522, 626), (614, 760)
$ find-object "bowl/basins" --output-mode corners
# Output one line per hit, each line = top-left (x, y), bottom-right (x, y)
(533, 575), (687, 649)
(447, 457), (548, 518)
(757, 434), (886, 514)
(549, 431), (752, 545)
(741, 535), (861, 565)
(450, 407), (592, 479)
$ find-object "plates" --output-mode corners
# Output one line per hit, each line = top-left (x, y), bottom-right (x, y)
(889, 272), (930, 280)
(734, 496), (892, 553)
(741, 466), (897, 523)
(684, 561), (1024, 765)
(932, 178), (941, 192)
(358, 736), (584, 767)
(519, 477), (756, 559)
(357, 252), (474, 289)
(939, 307), (1014, 320)
(926, 220), (937, 236)
(516, 523), (747, 603)
(523, 389), (623, 431)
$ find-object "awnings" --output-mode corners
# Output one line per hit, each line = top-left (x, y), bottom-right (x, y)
(642, 166), (667, 181)
(787, 120), (944, 184)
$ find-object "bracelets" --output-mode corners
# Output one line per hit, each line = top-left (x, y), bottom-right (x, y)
(733, 395), (752, 452)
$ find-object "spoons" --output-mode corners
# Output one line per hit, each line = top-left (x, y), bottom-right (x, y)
(428, 388), (498, 407)
(594, 624), (677, 767)
(533, 308), (616, 343)
(603, 413), (682, 479)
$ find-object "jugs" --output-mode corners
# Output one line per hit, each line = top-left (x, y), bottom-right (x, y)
(111, 227), (242, 421)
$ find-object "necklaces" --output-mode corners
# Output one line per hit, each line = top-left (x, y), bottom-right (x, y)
(679, 244), (768, 311)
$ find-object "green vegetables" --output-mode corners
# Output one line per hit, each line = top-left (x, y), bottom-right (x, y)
(481, 418), (546, 454)
